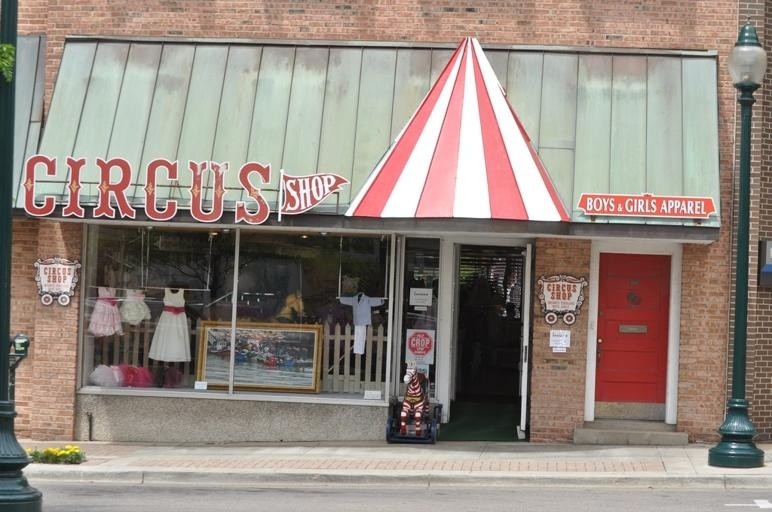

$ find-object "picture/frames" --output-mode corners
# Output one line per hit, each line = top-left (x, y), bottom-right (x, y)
(191, 319), (325, 394)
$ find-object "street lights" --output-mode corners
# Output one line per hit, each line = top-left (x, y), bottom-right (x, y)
(708, 22), (767, 468)
